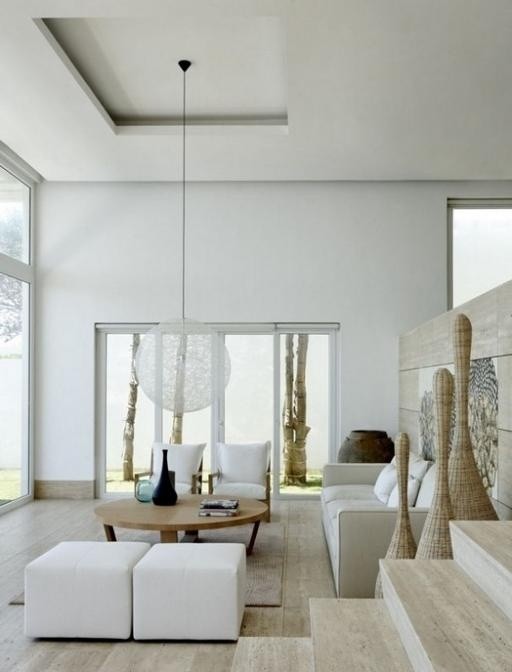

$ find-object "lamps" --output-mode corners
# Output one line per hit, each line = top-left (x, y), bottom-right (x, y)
(131, 59), (232, 414)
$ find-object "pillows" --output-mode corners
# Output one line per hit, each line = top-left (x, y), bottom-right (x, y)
(152, 439), (207, 484)
(373, 450), (437, 508)
(218, 440), (271, 486)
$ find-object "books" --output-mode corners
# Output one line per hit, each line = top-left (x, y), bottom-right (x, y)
(198, 498), (240, 517)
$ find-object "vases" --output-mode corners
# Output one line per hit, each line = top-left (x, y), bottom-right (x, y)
(337, 429), (394, 463)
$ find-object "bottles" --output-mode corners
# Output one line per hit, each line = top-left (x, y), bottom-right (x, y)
(135, 479), (155, 502)
(151, 449), (178, 506)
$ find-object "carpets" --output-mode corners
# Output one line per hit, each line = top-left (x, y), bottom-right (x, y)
(9, 520), (284, 607)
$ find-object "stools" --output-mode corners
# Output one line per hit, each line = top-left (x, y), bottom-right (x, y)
(132, 542), (248, 642)
(25, 541), (151, 641)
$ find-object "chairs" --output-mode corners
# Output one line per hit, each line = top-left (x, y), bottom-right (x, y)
(134, 449), (203, 494)
(208, 457), (271, 523)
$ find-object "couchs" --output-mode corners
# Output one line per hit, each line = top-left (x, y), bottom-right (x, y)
(319, 462), (429, 598)
